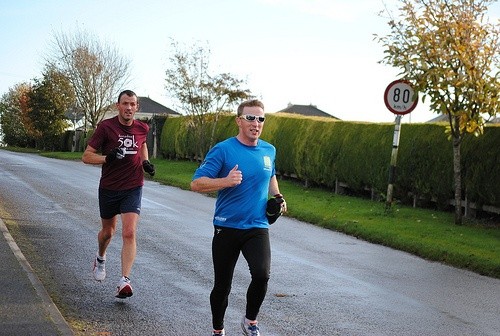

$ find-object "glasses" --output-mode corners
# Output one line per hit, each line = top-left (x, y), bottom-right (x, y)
(240, 115), (265, 124)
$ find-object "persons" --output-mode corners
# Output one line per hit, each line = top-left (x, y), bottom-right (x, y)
(81, 90), (155, 299)
(190, 99), (287, 336)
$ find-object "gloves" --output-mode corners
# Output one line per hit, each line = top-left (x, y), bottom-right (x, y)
(106, 147), (123, 163)
(142, 160), (155, 177)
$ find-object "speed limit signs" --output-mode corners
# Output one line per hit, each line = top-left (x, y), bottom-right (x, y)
(383, 80), (419, 115)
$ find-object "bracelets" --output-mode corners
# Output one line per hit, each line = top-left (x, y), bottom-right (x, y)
(274, 194), (283, 199)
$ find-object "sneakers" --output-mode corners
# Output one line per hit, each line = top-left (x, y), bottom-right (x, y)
(93, 250), (106, 281)
(240, 316), (260, 336)
(212, 328), (225, 336)
(115, 276), (133, 299)
